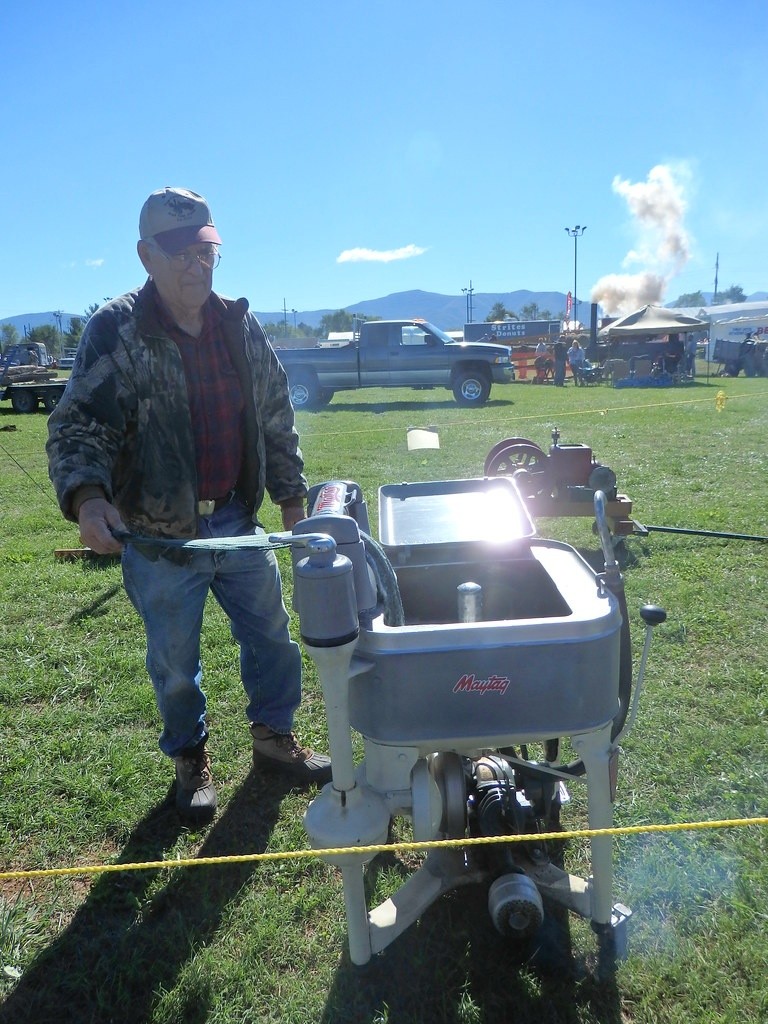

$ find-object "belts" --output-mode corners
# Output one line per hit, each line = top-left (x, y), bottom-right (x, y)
(196, 485), (244, 515)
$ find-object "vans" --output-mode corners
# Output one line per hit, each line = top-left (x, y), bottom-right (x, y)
(5, 342), (49, 367)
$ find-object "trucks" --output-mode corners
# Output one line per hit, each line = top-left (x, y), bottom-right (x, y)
(1, 380), (74, 414)
(711, 339), (768, 377)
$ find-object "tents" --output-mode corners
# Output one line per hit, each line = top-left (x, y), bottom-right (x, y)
(598, 303), (711, 386)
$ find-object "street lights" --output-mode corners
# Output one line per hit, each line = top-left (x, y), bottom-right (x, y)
(564, 225), (586, 331)
(291, 308), (297, 336)
(53, 310), (64, 358)
(461, 279), (476, 323)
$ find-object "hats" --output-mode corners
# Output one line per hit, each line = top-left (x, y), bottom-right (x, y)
(139, 186), (222, 256)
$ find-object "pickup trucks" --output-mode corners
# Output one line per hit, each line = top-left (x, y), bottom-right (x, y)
(271, 318), (513, 409)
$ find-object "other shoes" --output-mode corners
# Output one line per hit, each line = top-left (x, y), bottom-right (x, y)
(175, 749), (217, 818)
(249, 722), (332, 783)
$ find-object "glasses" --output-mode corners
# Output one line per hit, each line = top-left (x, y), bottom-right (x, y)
(147, 241), (221, 270)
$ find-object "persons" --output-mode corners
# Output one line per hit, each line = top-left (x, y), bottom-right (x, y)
(686, 335), (696, 377)
(535, 337), (547, 355)
(44, 185), (338, 828)
(554, 335), (569, 387)
(566, 339), (586, 387)
(27, 347), (39, 382)
(533, 352), (549, 385)
(753, 332), (761, 342)
(545, 355), (554, 378)
(744, 332), (752, 344)
